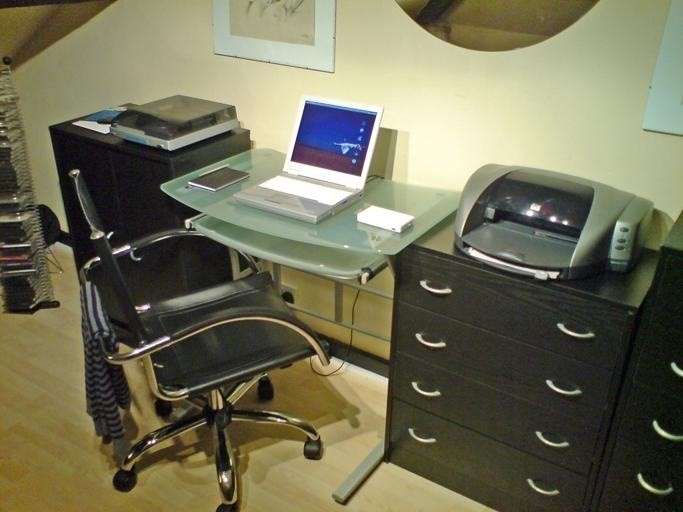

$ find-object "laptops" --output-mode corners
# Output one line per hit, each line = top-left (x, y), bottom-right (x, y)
(233, 96), (384, 226)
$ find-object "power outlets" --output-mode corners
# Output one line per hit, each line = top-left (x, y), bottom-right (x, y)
(280, 283), (297, 305)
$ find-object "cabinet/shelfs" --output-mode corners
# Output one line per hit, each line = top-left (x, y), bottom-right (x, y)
(47, 104), (258, 360)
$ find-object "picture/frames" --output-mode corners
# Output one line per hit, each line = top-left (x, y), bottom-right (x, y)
(212, 0), (337, 73)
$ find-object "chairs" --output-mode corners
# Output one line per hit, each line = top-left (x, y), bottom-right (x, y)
(68, 169), (333, 512)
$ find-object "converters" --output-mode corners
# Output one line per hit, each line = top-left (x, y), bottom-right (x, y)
(280, 291), (294, 308)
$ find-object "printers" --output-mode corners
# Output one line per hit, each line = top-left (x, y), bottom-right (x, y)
(454, 164), (656, 285)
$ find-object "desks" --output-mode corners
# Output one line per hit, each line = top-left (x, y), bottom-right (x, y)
(160, 148), (464, 505)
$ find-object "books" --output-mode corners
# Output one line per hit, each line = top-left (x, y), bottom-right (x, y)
(189, 166), (251, 193)
